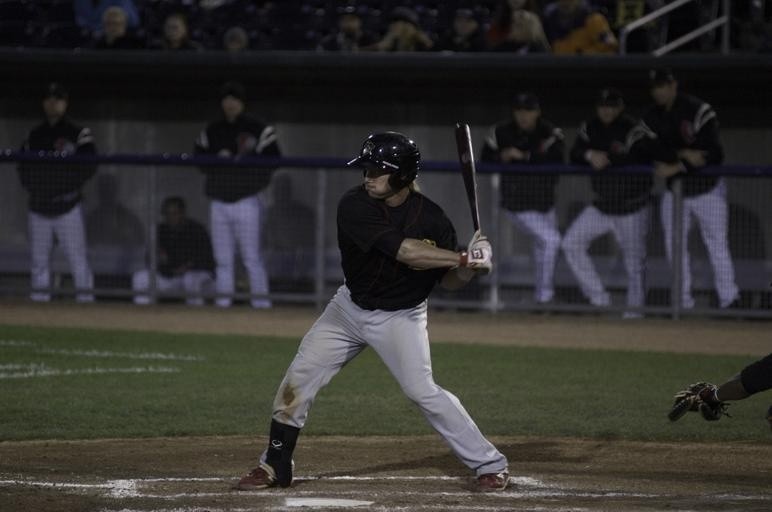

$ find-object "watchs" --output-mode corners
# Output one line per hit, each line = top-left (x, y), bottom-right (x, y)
(560, 87), (656, 320)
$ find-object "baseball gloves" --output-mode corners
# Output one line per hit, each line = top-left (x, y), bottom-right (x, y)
(668, 382), (730, 422)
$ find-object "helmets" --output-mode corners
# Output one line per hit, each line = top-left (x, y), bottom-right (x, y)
(217, 80), (244, 96)
(40, 82), (66, 98)
(595, 89), (620, 106)
(645, 68), (671, 84)
(347, 131), (420, 192)
(514, 94), (541, 110)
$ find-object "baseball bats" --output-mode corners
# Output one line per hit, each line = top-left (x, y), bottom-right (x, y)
(455, 124), (480, 232)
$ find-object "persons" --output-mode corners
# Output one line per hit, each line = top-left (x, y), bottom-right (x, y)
(129, 193), (214, 305)
(482, 93), (565, 300)
(669, 352), (772, 421)
(235, 128), (511, 487)
(18, 83), (101, 304)
(638, 67), (741, 308)
(198, 80), (283, 307)
(0, 2), (772, 56)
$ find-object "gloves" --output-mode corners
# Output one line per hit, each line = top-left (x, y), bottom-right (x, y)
(460, 231), (493, 275)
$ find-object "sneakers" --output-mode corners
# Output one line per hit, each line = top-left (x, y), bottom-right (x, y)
(472, 473), (509, 491)
(237, 457), (295, 491)
